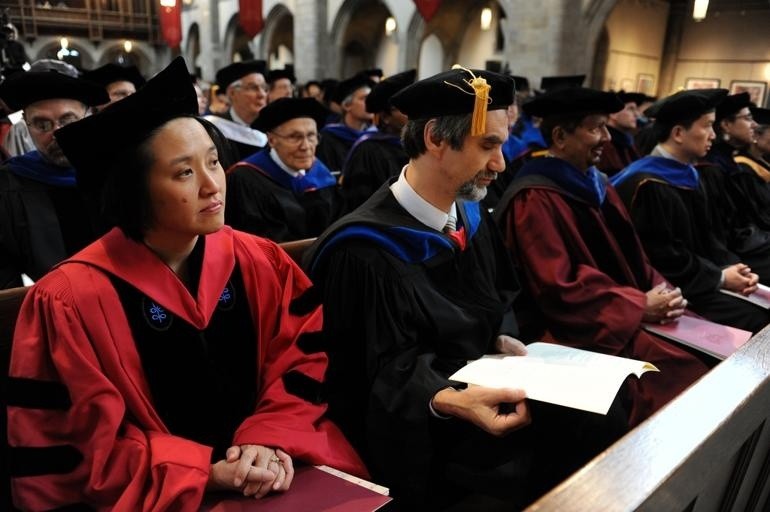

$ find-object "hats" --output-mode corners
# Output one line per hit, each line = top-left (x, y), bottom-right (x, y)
(1, 59), (111, 110)
(82, 61), (148, 90)
(50, 56), (198, 185)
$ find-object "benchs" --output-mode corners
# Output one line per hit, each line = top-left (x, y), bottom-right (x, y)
(0, 237), (317, 511)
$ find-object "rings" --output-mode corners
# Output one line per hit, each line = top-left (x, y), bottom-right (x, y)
(268, 455), (281, 463)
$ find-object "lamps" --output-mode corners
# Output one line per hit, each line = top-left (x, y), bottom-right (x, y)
(480, 7), (492, 28)
(693, 0), (709, 23)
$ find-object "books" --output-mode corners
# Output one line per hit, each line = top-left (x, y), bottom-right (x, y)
(210, 463), (393, 512)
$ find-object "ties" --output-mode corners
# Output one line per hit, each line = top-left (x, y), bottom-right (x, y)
(445, 216), (461, 236)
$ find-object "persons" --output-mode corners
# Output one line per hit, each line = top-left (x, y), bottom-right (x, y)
(305, 66), (634, 512)
(0, 57), (372, 511)
(0, 57), (769, 424)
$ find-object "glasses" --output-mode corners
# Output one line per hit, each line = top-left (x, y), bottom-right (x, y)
(275, 131), (321, 145)
(236, 83), (271, 93)
(27, 116), (81, 131)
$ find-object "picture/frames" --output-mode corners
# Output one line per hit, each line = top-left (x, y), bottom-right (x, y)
(684, 77), (721, 90)
(728, 80), (767, 108)
(636, 73), (655, 95)
